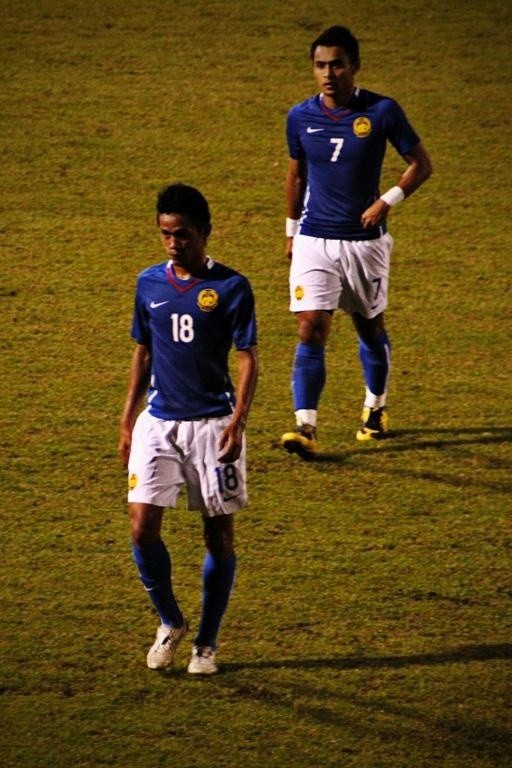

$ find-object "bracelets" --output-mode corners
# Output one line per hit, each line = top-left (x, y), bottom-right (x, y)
(379, 186), (406, 208)
(284, 216), (303, 238)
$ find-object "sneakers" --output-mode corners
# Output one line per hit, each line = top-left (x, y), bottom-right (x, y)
(188, 645), (218, 674)
(147, 619), (187, 669)
(356, 405), (389, 441)
(281, 423), (318, 460)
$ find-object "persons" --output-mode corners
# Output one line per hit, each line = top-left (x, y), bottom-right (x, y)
(277, 24), (431, 460)
(112, 181), (262, 679)
